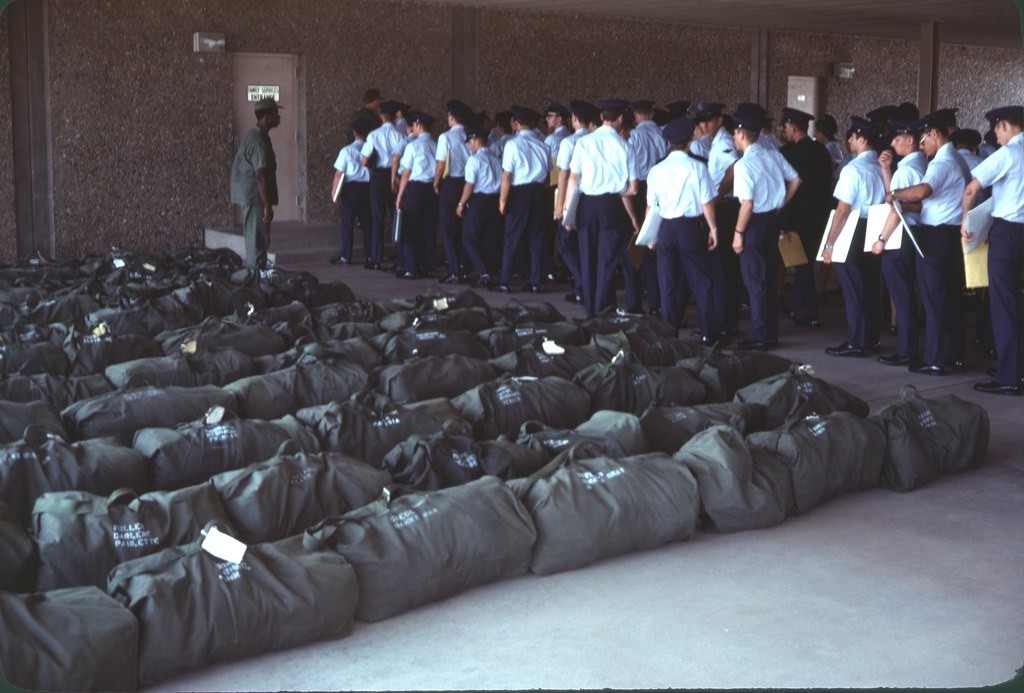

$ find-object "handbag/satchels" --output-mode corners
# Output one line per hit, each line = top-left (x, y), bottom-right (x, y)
(0, 241), (990, 693)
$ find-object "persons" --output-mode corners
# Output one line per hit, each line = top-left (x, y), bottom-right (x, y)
(231, 98), (284, 269)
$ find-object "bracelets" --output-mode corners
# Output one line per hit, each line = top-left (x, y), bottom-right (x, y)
(332, 85), (1023, 397)
(735, 230), (744, 234)
(458, 203), (463, 209)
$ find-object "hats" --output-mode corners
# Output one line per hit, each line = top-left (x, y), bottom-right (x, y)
(379, 99), (1024, 145)
(254, 97), (283, 110)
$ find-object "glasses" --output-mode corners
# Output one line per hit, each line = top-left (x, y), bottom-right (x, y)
(919, 133), (929, 144)
(545, 113), (558, 118)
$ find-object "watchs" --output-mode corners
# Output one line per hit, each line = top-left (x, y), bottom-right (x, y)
(891, 189), (899, 201)
(823, 243), (835, 251)
(877, 233), (886, 244)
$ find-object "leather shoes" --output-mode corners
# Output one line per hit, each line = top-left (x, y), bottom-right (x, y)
(487, 283), (510, 293)
(689, 331), (713, 346)
(876, 355), (911, 365)
(794, 320), (818, 329)
(910, 365), (943, 376)
(565, 294), (583, 304)
(470, 277), (488, 287)
(384, 264), (399, 273)
(529, 286), (539, 294)
(976, 339), (997, 360)
(826, 344), (879, 357)
(649, 309), (658, 316)
(459, 273), (472, 283)
(426, 272), (433, 278)
(438, 271), (458, 284)
(738, 334), (779, 351)
(397, 271), (415, 278)
(717, 328), (737, 337)
(974, 368), (1024, 394)
(890, 326), (898, 336)
(364, 262), (375, 269)
(544, 274), (557, 281)
(332, 258), (348, 266)
